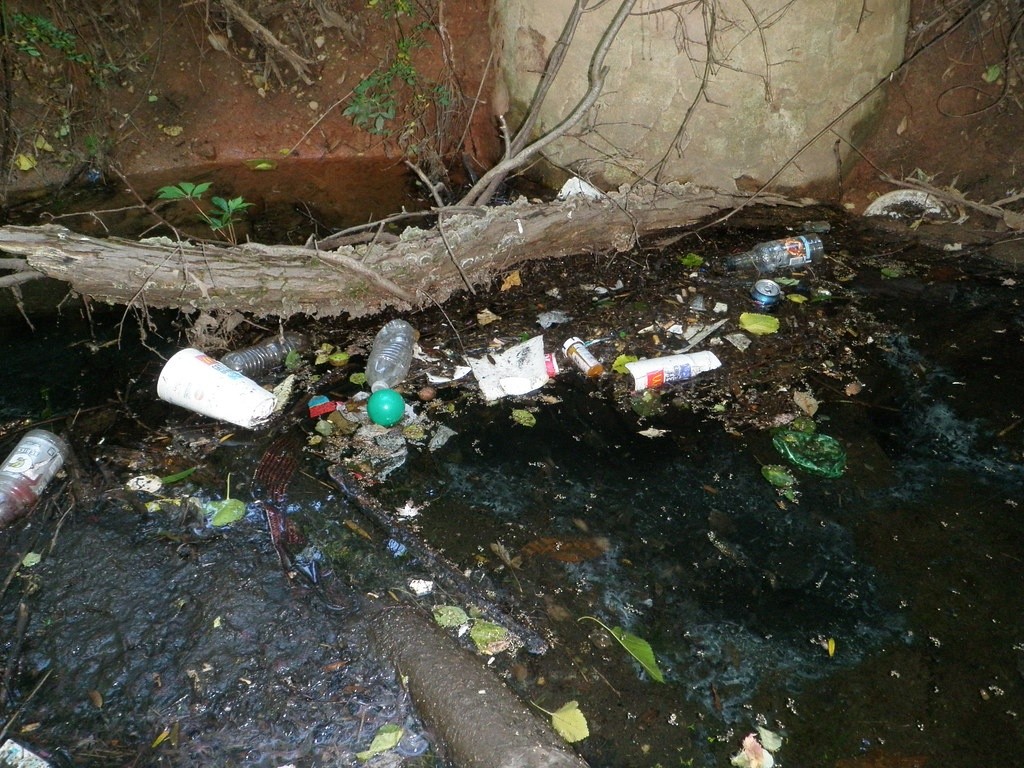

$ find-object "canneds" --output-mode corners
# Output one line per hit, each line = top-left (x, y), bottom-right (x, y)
(750, 279), (781, 304)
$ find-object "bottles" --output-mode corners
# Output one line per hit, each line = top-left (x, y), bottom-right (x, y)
(730, 233), (823, 273)
(365, 319), (415, 394)
(0, 429), (69, 526)
(220, 332), (309, 376)
(0, 738), (57, 768)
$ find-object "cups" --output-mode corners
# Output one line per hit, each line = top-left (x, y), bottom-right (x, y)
(157, 348), (278, 429)
